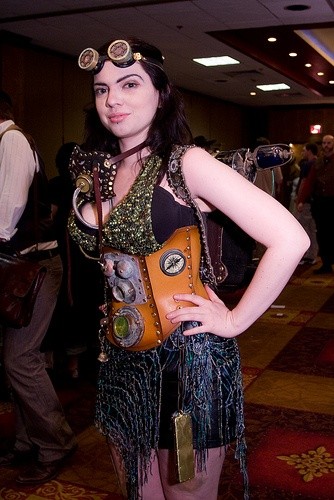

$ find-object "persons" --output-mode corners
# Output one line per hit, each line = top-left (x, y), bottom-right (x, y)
(0, 90), (78, 484)
(50, 135), (334, 382)
(67, 37), (311, 500)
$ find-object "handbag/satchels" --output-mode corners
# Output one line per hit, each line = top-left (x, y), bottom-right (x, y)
(0, 253), (47, 329)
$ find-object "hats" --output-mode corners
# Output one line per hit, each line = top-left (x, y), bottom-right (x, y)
(194, 136), (217, 146)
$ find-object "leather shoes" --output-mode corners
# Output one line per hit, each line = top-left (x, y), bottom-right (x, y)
(0, 444), (25, 468)
(16, 444), (79, 487)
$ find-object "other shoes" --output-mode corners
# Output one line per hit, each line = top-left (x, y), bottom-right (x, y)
(314, 265), (331, 273)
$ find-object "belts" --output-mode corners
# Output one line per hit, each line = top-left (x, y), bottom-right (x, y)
(19, 247), (61, 261)
